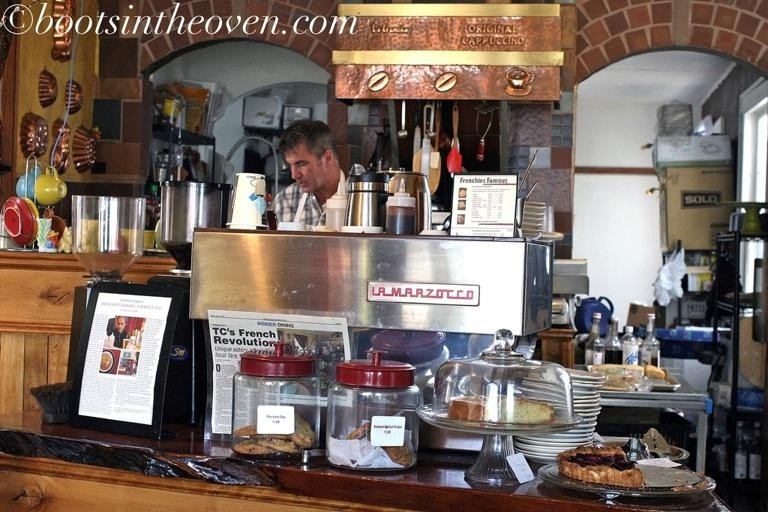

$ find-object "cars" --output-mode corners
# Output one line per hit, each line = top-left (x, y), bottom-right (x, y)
(44, 216), (68, 241)
(227, 171), (271, 232)
(14, 160), (65, 206)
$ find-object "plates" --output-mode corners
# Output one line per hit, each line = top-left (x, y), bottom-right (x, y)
(701, 235), (768, 492)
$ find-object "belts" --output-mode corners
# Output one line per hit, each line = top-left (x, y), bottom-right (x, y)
(417, 403), (582, 489)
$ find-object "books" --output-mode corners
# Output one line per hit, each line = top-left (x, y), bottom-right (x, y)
(651, 131), (736, 343)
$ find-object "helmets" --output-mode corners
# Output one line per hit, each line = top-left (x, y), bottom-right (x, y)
(582, 442), (690, 463)
(522, 200), (547, 239)
(508, 363), (608, 465)
(586, 363), (682, 392)
(538, 461), (719, 498)
(3, 198), (42, 248)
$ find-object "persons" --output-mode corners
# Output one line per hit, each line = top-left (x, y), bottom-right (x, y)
(109, 316), (129, 349)
(267, 118), (354, 226)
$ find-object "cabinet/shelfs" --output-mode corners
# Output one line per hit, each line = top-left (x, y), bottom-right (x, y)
(325, 348), (422, 471)
(712, 416), (764, 484)
(386, 171), (433, 234)
(386, 177), (416, 235)
(230, 338), (319, 455)
(367, 322), (450, 382)
(347, 171), (387, 231)
(324, 182), (348, 228)
(584, 311), (661, 367)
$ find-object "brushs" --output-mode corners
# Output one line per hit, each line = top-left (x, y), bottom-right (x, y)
(67, 194), (147, 392)
(146, 179), (228, 431)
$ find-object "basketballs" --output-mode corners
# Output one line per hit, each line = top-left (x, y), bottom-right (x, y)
(231, 413), (413, 466)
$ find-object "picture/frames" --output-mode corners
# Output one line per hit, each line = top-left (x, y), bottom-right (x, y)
(573, 376), (712, 485)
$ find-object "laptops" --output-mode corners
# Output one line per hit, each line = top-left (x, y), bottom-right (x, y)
(448, 394), (555, 425)
(556, 447), (644, 489)
(592, 363), (666, 386)
